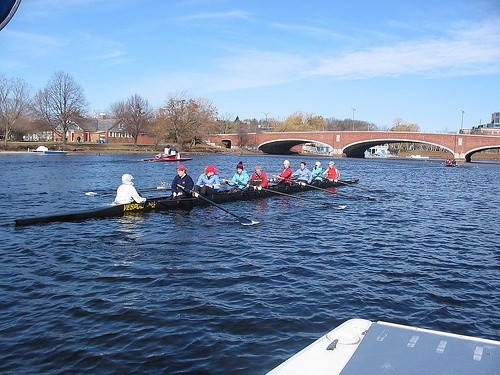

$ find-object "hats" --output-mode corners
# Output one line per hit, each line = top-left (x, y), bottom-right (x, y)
(237, 161), (244, 169)
(328, 160), (336, 165)
(176, 165), (187, 170)
(315, 161), (321, 167)
(206, 165), (218, 173)
(284, 160), (290, 165)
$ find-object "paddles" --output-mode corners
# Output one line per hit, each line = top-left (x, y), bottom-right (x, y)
(85, 184), (204, 196)
(271, 174), (378, 201)
(239, 183), (346, 209)
(320, 176), (386, 192)
(267, 178), (278, 182)
(176, 183), (252, 223)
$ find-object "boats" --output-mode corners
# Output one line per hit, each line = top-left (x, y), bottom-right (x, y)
(142, 154), (192, 163)
(13, 176), (360, 225)
(32, 145), (69, 155)
(442, 160), (457, 166)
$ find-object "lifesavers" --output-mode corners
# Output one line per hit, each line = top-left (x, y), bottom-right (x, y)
(155, 155), (160, 159)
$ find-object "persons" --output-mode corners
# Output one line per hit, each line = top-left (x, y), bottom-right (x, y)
(311, 161), (323, 182)
(445, 158), (455, 164)
(196, 165), (220, 191)
(277, 159), (293, 185)
(322, 161), (340, 182)
(77, 135), (81, 142)
(222, 161), (249, 190)
(114, 174), (147, 205)
(250, 166), (268, 190)
(294, 161), (313, 184)
(171, 165), (194, 198)
